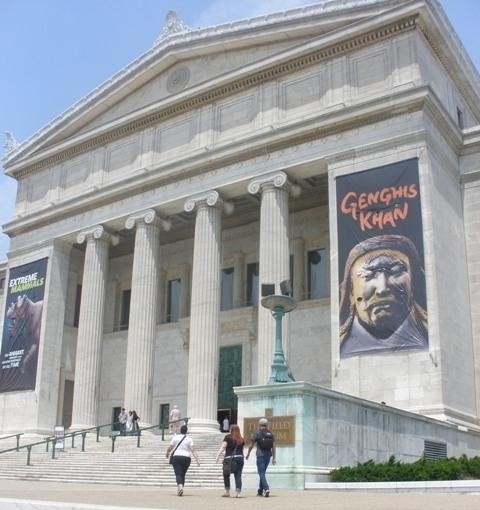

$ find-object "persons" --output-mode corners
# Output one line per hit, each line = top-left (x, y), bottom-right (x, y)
(165, 425), (200, 496)
(215, 424), (245, 498)
(118, 408), (141, 436)
(169, 405), (181, 435)
(339, 234), (429, 357)
(222, 416), (230, 433)
(246, 418), (276, 497)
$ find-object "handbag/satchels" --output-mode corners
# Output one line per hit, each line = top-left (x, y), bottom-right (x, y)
(222, 463), (232, 474)
(168, 455), (174, 465)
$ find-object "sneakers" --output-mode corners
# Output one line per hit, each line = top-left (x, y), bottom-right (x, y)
(256, 490), (271, 498)
(222, 493), (231, 498)
(177, 489), (184, 496)
(235, 493), (242, 498)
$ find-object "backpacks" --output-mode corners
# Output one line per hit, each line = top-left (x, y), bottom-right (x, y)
(256, 429), (275, 449)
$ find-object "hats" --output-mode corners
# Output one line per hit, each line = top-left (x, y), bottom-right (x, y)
(258, 418), (269, 426)
